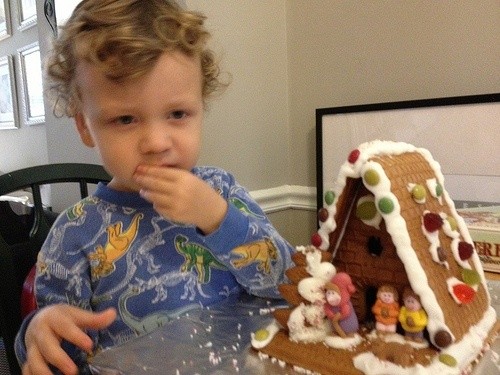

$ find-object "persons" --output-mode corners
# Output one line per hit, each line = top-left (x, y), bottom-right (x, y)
(15, 1), (300, 375)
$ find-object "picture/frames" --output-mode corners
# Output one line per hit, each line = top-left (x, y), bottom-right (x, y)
(17, 41), (46, 126)
(14, 0), (37, 32)
(0, 0), (12, 42)
(0, 54), (22, 130)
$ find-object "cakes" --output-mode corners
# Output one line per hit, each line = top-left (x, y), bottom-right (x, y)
(248, 139), (500, 375)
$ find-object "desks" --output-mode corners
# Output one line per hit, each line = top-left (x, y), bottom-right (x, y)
(87, 280), (500, 375)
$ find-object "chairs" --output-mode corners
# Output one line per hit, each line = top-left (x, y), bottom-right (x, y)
(0, 163), (113, 375)
